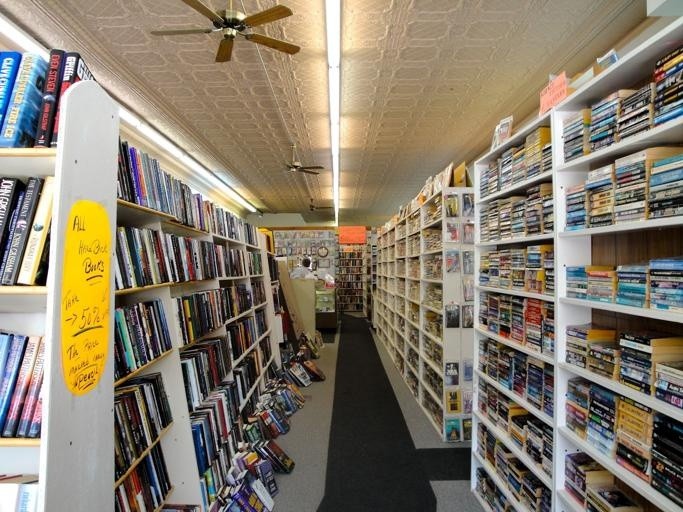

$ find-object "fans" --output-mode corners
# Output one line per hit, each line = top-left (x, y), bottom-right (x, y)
(140, 2), (302, 68)
(280, 149), (324, 180)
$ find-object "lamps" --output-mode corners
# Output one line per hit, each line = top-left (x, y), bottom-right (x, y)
(327, 77), (342, 228)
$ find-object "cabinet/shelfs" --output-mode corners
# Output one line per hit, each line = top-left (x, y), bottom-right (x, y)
(471, 18), (682, 508)
(371, 186), (474, 445)
(338, 243), (362, 313)
(4, 74), (294, 512)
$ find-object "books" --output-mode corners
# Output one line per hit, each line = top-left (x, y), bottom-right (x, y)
(274, 232), (376, 316)
(376, 51), (683, 511)
(116, 139), (325, 511)
(0, 50), (97, 511)
